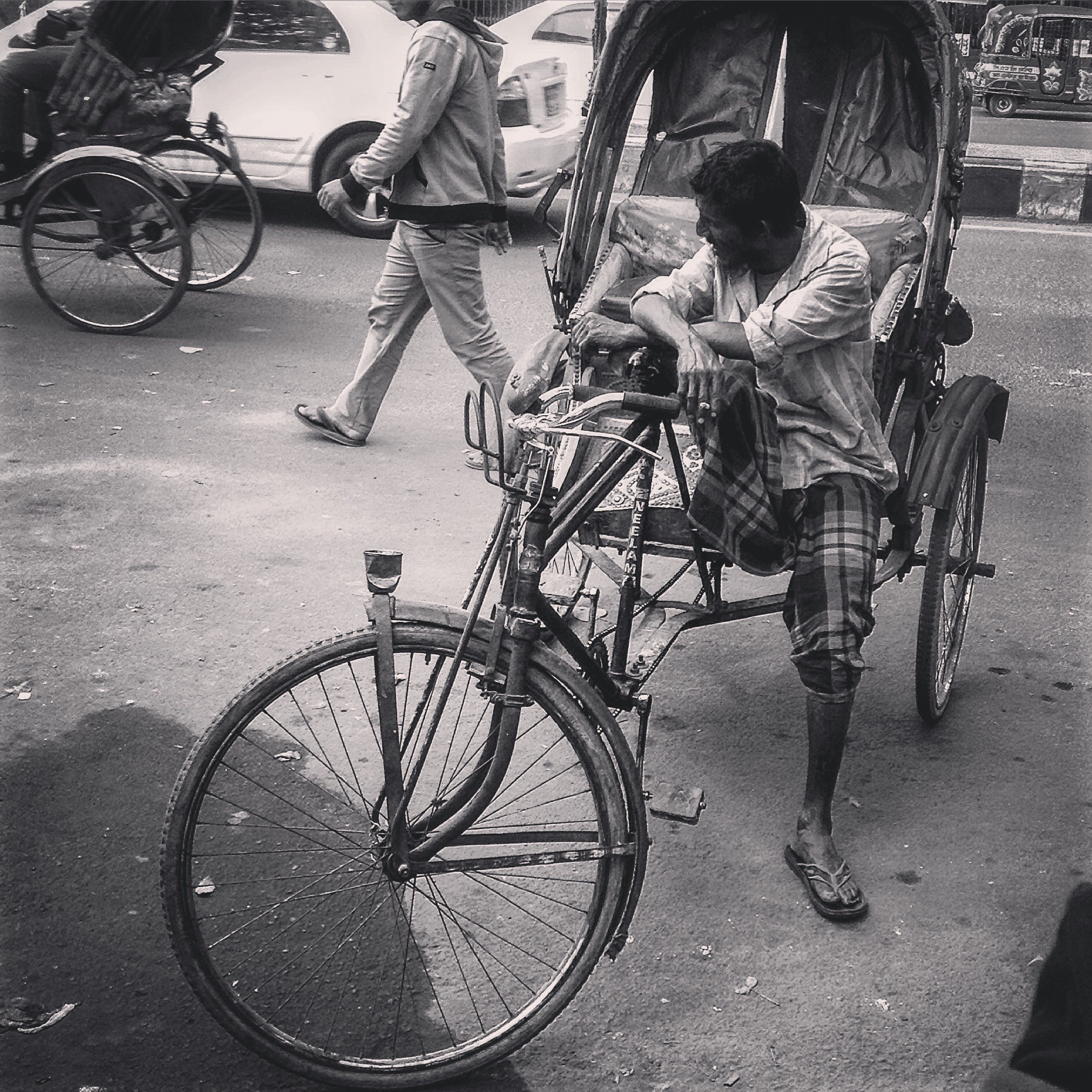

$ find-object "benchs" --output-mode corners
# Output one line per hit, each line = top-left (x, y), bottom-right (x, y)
(566, 195), (927, 391)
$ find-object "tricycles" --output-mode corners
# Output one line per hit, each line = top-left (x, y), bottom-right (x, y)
(0, 1), (267, 334)
(158, 2), (1010, 1092)
(969, 4), (1092, 118)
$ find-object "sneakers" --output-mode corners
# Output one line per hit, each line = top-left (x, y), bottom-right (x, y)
(0, 151), (34, 186)
(31, 134), (59, 164)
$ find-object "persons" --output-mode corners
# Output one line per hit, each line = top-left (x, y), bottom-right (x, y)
(0, 44), (78, 182)
(630, 141), (900, 924)
(293, 3), (551, 469)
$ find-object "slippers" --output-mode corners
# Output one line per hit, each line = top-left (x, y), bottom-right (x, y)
(466, 451), (545, 469)
(294, 403), (368, 448)
(785, 841), (870, 920)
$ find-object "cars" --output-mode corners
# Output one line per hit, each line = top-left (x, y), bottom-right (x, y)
(1, 1), (584, 239)
(484, 0), (655, 126)
